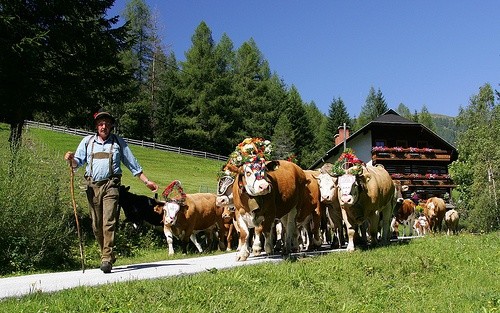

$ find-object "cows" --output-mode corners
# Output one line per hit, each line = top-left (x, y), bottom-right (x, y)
(154, 159), (460, 262)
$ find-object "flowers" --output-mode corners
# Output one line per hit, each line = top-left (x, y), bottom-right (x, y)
(330, 145), (366, 177)
(161, 180), (187, 204)
(216, 137), (275, 182)
(372, 145), (450, 180)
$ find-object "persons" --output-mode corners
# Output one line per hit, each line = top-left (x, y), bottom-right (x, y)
(65, 111), (158, 273)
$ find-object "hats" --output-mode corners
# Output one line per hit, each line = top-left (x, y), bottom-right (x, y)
(93, 111), (115, 125)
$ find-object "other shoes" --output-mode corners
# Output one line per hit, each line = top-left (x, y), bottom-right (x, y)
(101, 261), (111, 274)
(111, 255), (116, 264)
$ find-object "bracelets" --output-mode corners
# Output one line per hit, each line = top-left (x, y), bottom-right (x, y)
(144, 179), (149, 184)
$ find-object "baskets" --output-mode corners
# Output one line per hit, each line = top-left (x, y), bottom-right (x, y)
(372, 146), (436, 158)
(391, 173), (452, 186)
